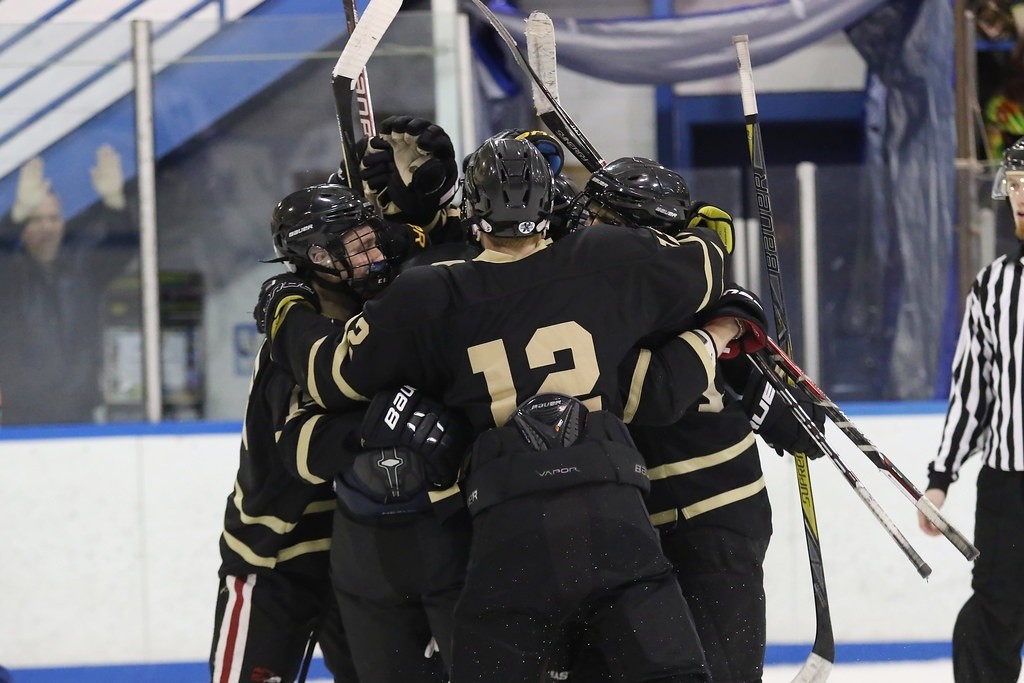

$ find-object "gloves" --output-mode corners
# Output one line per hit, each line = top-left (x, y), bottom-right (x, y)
(707, 281), (769, 361)
(357, 136), (447, 233)
(254, 271), (322, 346)
(378, 113), (461, 210)
(327, 136), (412, 218)
(748, 382), (826, 460)
(684, 198), (735, 257)
(494, 127), (564, 178)
(358, 380), (474, 490)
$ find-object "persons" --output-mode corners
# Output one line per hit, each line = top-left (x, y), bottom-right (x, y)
(208, 115), (826, 683)
(0, 143), (134, 427)
(916, 137), (1024, 683)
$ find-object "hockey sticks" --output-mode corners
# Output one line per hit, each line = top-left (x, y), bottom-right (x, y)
(339, 0), (378, 141)
(523, 7), (986, 565)
(729, 30), (837, 683)
(328, 0), (405, 197)
(471, 0), (935, 585)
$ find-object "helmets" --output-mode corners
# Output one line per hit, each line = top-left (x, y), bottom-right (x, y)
(459, 132), (555, 239)
(271, 182), (400, 297)
(998, 138), (1023, 171)
(549, 172), (585, 234)
(563, 157), (694, 236)
